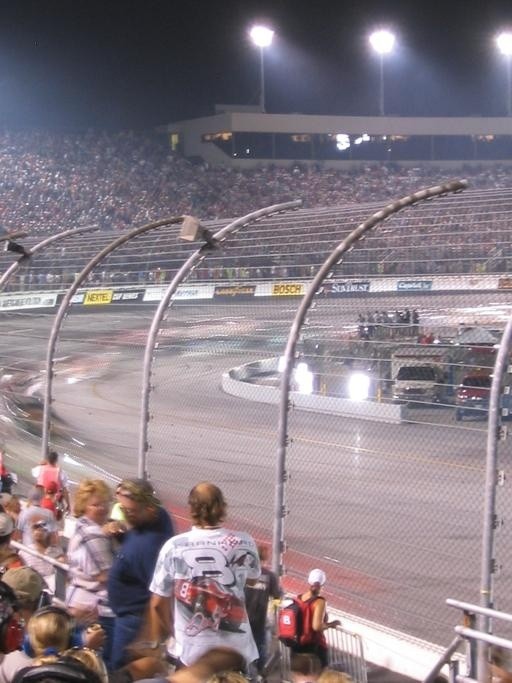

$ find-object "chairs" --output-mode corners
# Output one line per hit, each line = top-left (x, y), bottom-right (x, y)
(12, 659), (102, 683)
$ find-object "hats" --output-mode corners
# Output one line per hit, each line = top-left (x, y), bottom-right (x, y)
(309, 568), (326, 585)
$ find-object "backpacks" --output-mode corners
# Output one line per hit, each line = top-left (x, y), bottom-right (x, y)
(278, 593), (324, 647)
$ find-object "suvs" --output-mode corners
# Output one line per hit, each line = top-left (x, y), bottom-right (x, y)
(450, 369), (503, 422)
(384, 363), (448, 408)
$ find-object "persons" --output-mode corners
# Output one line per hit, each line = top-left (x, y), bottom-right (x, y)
(1, 447), (355, 683)
(356, 308), (466, 346)
(2, 126), (511, 292)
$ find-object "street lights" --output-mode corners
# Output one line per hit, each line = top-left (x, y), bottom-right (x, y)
(494, 29), (512, 120)
(248, 22), (275, 112)
(365, 29), (396, 115)
